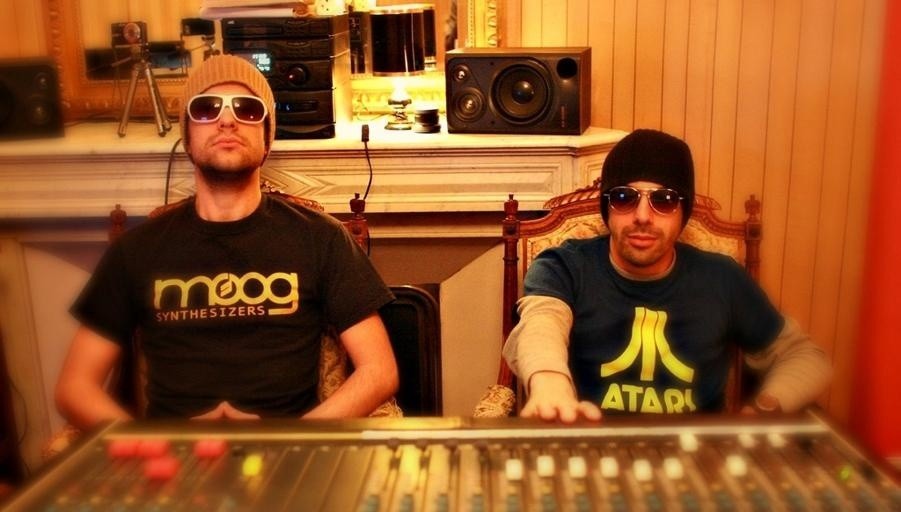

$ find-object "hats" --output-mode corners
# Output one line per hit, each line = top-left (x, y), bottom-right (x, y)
(178, 54), (277, 166)
(599, 128), (695, 231)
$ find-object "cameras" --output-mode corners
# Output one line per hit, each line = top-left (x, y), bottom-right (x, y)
(182, 18), (215, 36)
(111, 21), (148, 48)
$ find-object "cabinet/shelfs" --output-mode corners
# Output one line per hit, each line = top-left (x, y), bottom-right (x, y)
(3, 115), (635, 445)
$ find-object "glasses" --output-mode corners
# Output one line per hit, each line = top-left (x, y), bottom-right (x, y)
(186, 94), (269, 125)
(603, 186), (684, 217)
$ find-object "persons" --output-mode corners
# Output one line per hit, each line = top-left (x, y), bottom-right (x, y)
(53, 50), (409, 433)
(503, 129), (845, 422)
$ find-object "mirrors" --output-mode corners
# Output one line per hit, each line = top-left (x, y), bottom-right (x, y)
(38, 0), (504, 124)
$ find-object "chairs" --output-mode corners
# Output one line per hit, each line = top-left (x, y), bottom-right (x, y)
(476, 174), (768, 419)
(106, 191), (373, 432)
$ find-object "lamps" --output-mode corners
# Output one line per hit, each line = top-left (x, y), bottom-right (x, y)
(366, 5), (427, 130)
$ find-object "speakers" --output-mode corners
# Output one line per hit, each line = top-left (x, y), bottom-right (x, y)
(445, 46), (592, 136)
(0, 61), (61, 138)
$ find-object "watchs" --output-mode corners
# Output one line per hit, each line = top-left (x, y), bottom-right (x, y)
(747, 393), (786, 414)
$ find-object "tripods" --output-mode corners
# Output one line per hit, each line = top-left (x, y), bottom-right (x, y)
(110, 47), (172, 138)
